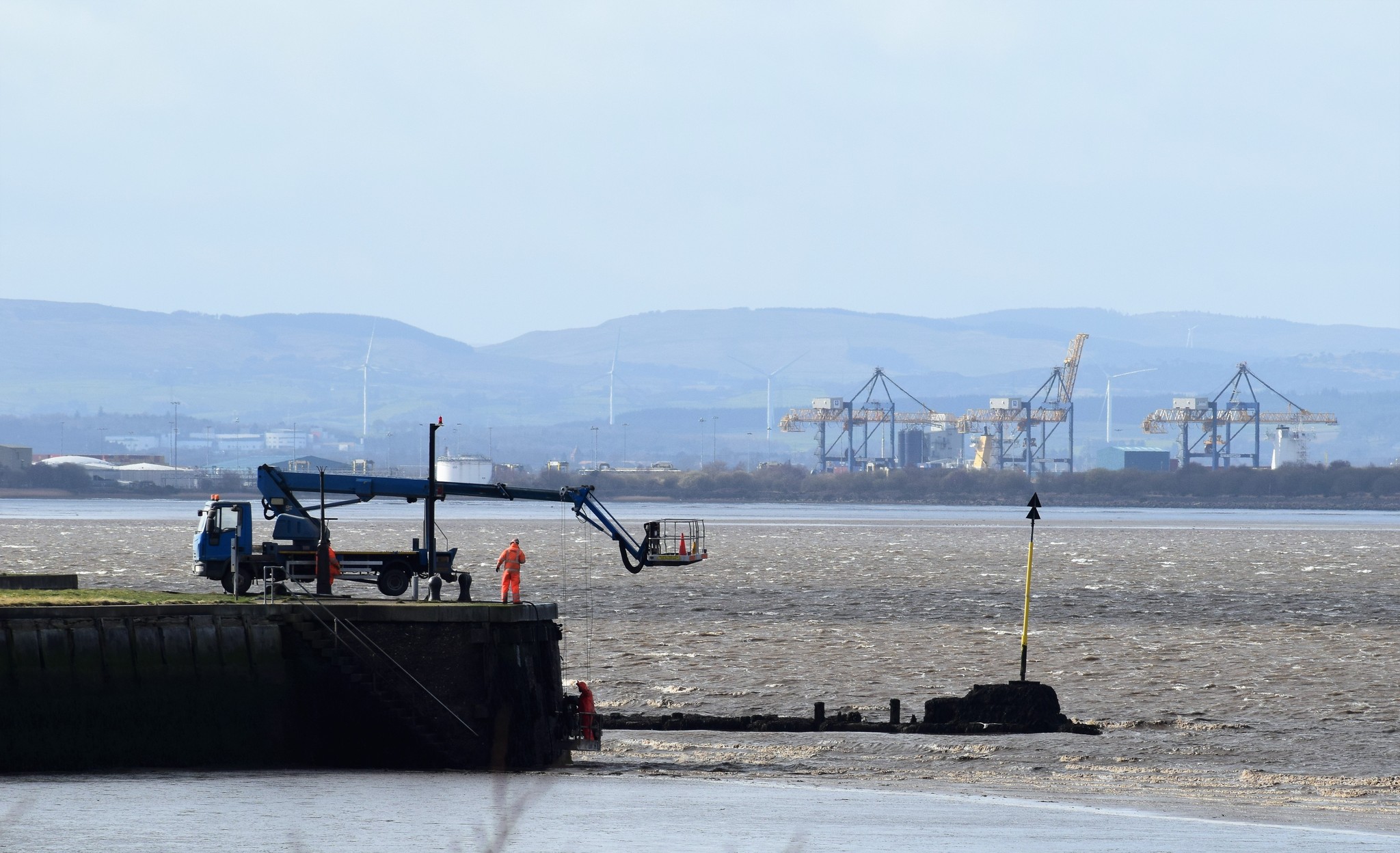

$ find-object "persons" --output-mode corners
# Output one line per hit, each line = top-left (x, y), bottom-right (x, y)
(576, 680), (595, 740)
(496, 538), (525, 604)
(316, 539), (341, 595)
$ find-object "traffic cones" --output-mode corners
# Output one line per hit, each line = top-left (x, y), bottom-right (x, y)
(692, 542), (696, 555)
(678, 533), (687, 556)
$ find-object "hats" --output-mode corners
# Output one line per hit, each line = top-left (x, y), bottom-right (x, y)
(325, 539), (330, 543)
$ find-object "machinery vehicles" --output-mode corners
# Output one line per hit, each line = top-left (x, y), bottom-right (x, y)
(192, 464), (708, 595)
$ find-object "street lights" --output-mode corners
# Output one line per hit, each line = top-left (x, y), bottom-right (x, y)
(386, 432), (393, 475)
(169, 402), (180, 485)
(128, 431), (133, 455)
(488, 427), (493, 459)
(235, 418), (240, 472)
(591, 426), (599, 470)
(207, 426), (211, 466)
(453, 423), (461, 455)
(699, 418), (706, 470)
(419, 424), (423, 476)
(748, 433), (753, 473)
(61, 422), (65, 456)
(294, 423), (296, 462)
(96, 428), (108, 455)
(622, 423), (629, 467)
(767, 428), (772, 463)
(712, 417), (719, 462)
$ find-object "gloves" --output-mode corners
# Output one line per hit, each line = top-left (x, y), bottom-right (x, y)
(576, 680), (580, 686)
(336, 564), (341, 570)
(496, 567), (500, 572)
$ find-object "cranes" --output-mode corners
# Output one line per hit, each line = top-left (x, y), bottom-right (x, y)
(1141, 360), (1337, 476)
(970, 333), (1090, 484)
(781, 367), (983, 475)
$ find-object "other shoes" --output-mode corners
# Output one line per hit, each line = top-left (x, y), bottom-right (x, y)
(513, 601), (523, 605)
(502, 601), (508, 604)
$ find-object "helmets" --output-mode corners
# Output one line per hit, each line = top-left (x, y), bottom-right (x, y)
(511, 538), (520, 545)
